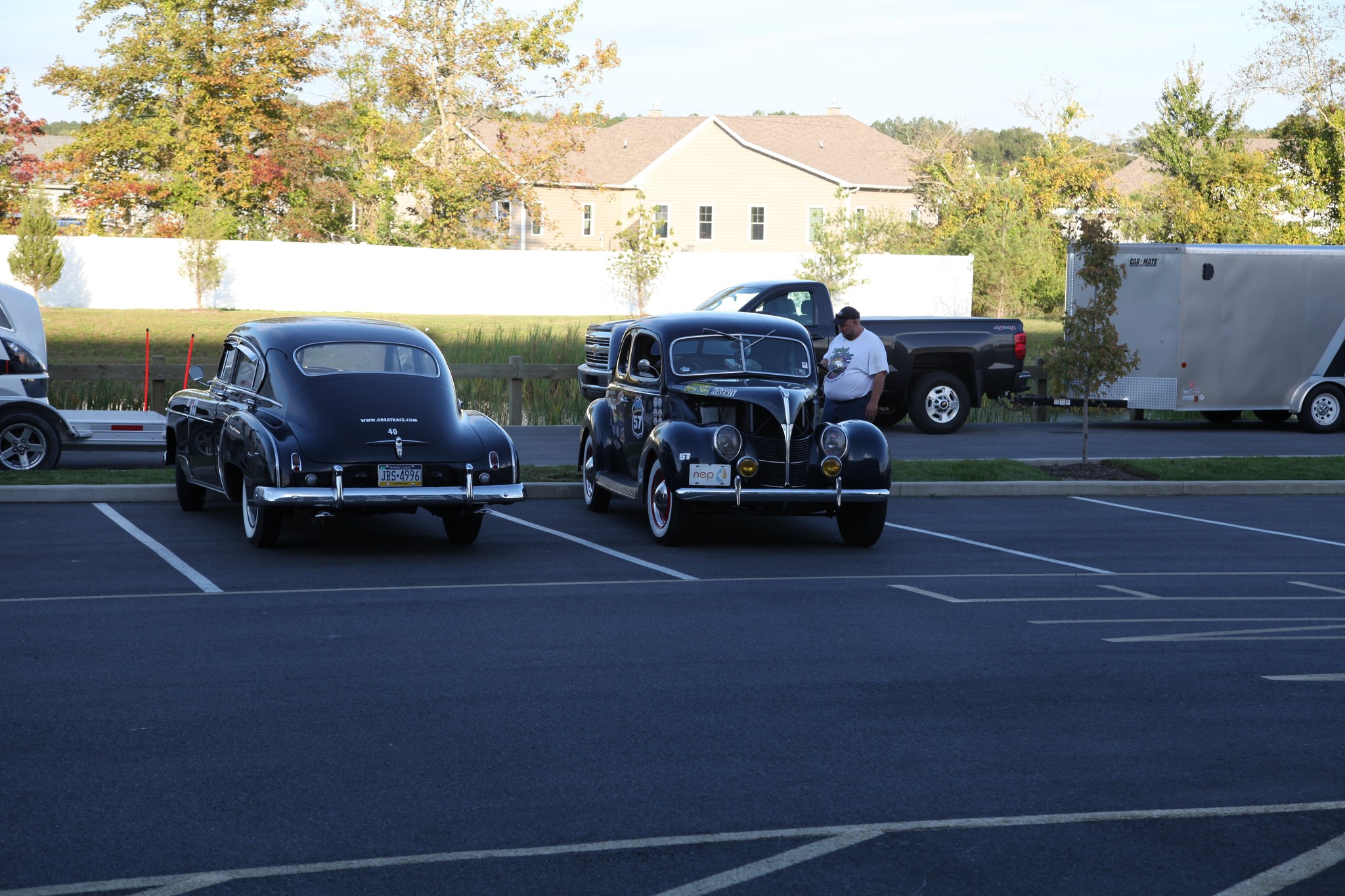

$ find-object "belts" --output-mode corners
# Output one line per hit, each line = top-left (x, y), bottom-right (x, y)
(831, 392), (871, 403)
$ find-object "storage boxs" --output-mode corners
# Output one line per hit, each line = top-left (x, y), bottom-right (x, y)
(724, 358), (761, 371)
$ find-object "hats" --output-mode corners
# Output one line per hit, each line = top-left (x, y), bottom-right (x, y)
(831, 306), (860, 325)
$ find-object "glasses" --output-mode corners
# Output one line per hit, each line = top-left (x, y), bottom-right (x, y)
(835, 312), (856, 321)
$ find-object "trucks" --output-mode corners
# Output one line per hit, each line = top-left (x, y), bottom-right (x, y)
(1066, 236), (1343, 431)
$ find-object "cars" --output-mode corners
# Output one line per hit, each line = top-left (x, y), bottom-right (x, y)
(166, 314), (527, 549)
(583, 313), (897, 547)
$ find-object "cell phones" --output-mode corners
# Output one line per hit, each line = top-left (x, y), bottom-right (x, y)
(816, 363), (831, 373)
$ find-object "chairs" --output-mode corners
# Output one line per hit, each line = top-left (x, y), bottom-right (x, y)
(797, 300), (813, 325)
(775, 299), (798, 322)
(651, 356), (703, 377)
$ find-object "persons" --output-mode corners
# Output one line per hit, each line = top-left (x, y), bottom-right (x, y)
(816, 306), (890, 429)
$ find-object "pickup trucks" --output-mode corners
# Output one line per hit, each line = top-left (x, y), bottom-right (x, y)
(573, 277), (1030, 434)
